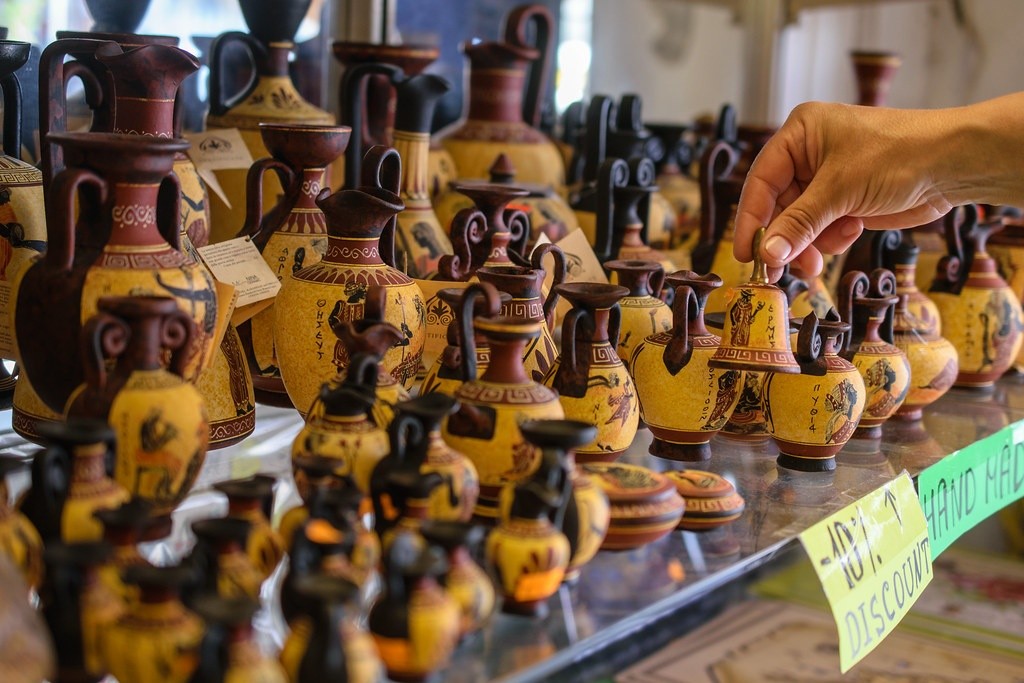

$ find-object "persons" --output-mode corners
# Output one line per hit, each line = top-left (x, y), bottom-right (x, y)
(733, 86), (1024, 280)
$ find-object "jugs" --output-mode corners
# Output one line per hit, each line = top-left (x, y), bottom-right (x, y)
(0, 0), (1024, 683)
